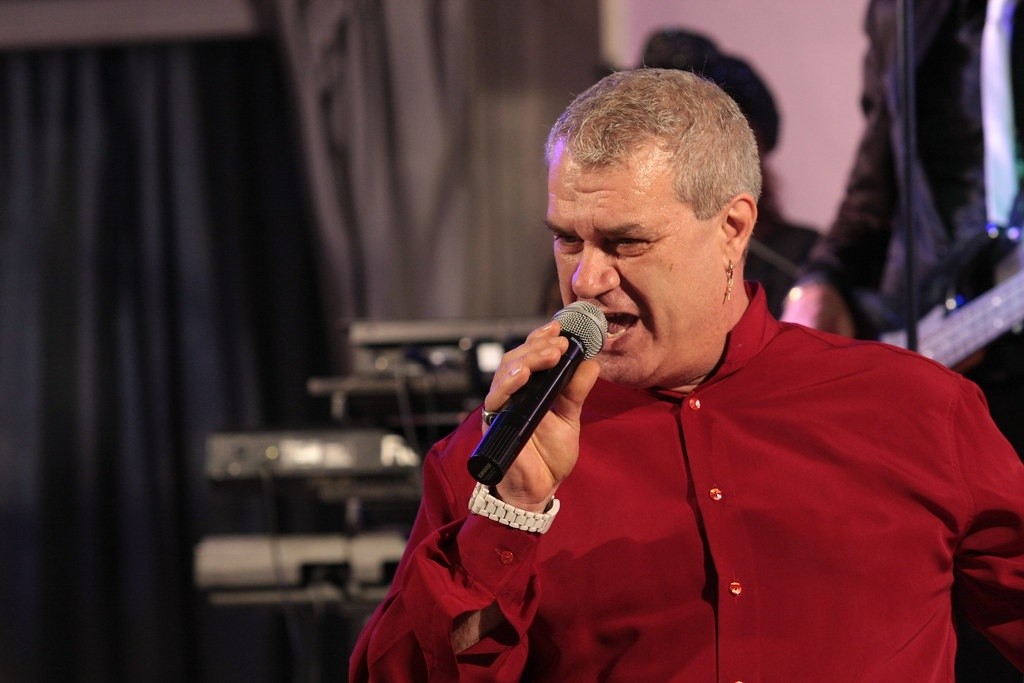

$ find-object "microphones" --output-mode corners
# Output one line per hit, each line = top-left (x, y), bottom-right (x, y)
(468, 302), (609, 486)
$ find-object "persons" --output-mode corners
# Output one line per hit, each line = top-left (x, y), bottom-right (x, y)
(593, 28), (902, 335)
(349, 67), (1024, 683)
(781, 0), (1024, 683)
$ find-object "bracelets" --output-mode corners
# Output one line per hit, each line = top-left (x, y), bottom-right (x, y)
(467, 482), (561, 534)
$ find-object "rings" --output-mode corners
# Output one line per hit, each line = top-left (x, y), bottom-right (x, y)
(481, 408), (497, 426)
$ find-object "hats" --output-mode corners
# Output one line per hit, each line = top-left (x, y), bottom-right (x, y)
(642, 31), (782, 151)
